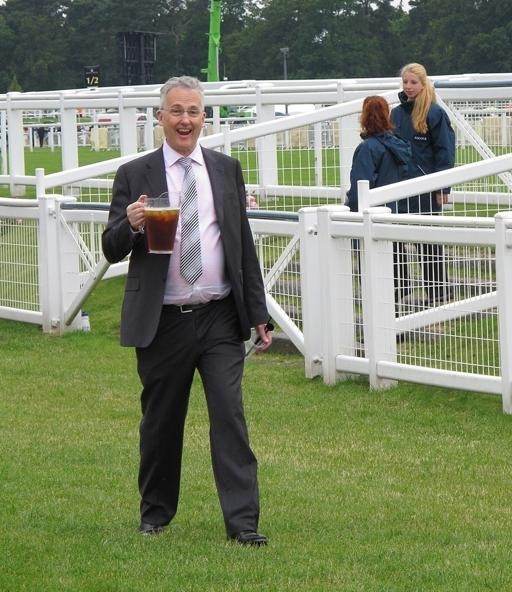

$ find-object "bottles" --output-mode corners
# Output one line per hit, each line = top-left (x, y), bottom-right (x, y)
(81, 312), (90, 331)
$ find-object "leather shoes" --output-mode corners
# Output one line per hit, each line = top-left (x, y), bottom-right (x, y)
(225, 531), (267, 544)
(136, 520), (166, 535)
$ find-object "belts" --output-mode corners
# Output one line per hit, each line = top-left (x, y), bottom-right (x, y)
(179, 303), (210, 314)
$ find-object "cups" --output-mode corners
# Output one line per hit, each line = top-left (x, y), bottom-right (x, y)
(136, 197), (182, 256)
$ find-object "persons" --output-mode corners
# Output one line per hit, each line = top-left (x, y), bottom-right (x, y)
(390, 64), (455, 302)
(349, 96), (410, 341)
(101, 74), (276, 543)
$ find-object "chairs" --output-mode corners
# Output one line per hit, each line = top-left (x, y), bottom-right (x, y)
(21, 113), (512, 155)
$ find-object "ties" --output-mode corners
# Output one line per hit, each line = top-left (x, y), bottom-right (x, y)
(179, 157), (202, 285)
